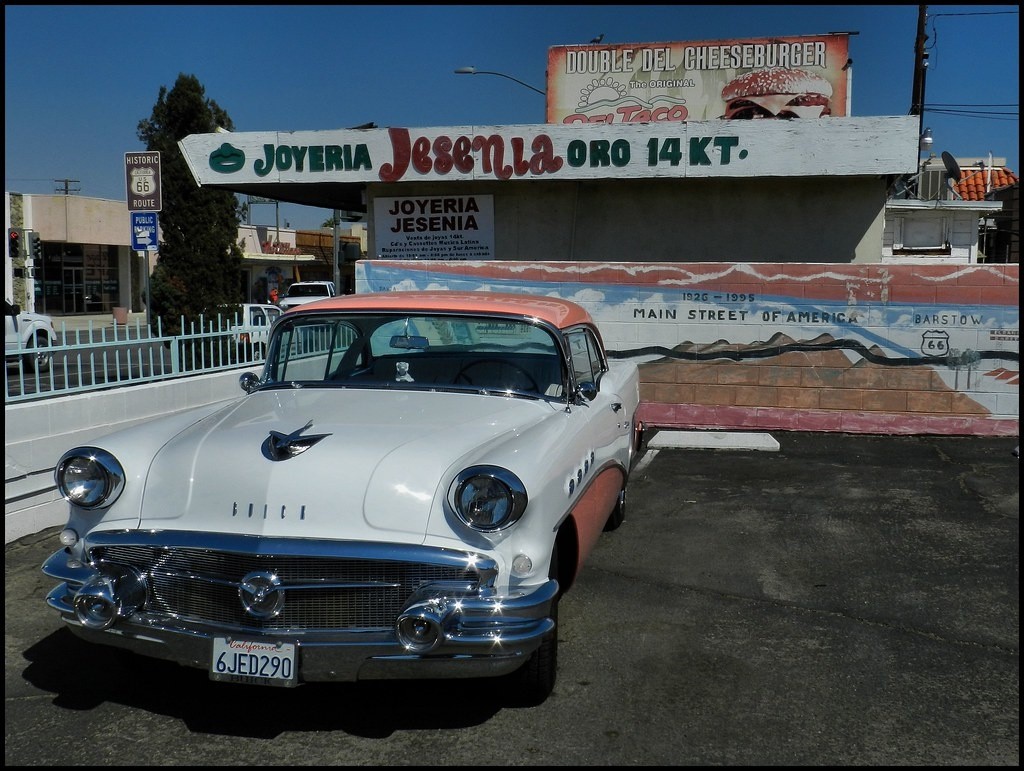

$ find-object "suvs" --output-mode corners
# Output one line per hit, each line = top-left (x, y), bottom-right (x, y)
(190, 302), (307, 364)
(275, 280), (346, 317)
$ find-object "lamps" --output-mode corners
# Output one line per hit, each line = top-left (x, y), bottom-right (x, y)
(921, 127), (933, 152)
(454, 67), (545, 95)
(927, 151), (936, 163)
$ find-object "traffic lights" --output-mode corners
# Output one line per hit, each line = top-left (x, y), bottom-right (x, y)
(28, 231), (42, 259)
(7, 228), (22, 258)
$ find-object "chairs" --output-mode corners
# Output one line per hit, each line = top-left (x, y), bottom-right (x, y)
(253, 315), (265, 326)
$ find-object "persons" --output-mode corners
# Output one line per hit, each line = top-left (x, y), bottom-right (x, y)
(269, 287), (278, 301)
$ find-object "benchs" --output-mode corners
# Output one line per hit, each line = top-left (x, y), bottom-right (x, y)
(370, 353), (565, 400)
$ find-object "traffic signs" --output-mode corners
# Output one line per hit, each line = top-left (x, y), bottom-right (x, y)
(132, 212), (159, 251)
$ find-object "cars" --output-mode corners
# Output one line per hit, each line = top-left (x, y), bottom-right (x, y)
(40, 290), (649, 706)
(4, 298), (59, 374)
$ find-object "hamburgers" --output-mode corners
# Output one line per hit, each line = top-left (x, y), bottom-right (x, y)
(715, 68), (833, 119)
